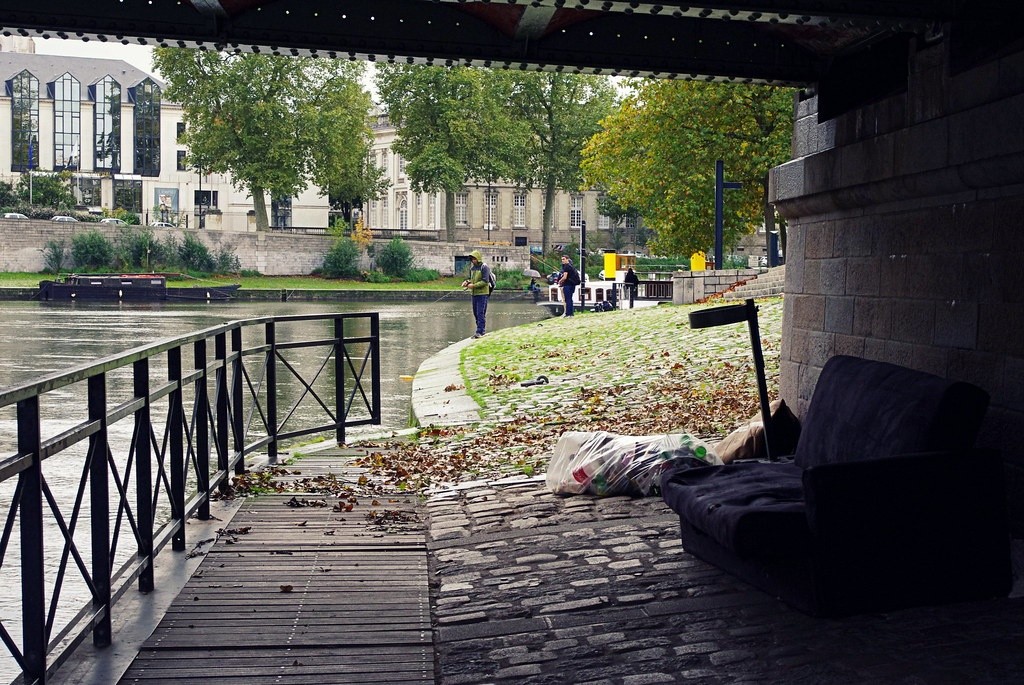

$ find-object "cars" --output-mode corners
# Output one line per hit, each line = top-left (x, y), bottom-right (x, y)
(547, 269), (590, 285)
(150, 221), (176, 228)
(101, 218), (128, 226)
(3, 213), (29, 220)
(51, 215), (79, 222)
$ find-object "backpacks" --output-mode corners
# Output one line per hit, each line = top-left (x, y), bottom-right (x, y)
(487, 272), (497, 297)
(593, 303), (602, 312)
(603, 301), (616, 312)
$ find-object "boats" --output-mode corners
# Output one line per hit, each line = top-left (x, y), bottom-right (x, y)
(536, 270), (661, 318)
(38, 272), (242, 303)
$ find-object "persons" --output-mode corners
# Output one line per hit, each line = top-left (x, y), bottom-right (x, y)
(556, 255), (581, 318)
(461, 250), (490, 340)
(531, 277), (536, 290)
(624, 268), (640, 290)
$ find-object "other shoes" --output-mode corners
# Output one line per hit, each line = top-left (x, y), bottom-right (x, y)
(564, 315), (574, 319)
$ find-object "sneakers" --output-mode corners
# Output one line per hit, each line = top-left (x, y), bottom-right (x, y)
(471, 332), (482, 339)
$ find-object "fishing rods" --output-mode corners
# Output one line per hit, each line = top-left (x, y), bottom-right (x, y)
(424, 281), (466, 307)
(498, 291), (536, 303)
(515, 246), (562, 274)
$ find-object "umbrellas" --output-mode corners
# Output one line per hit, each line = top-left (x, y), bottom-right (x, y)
(522, 270), (541, 278)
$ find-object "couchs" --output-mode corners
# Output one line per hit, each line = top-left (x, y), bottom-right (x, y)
(660, 355), (1013, 621)
